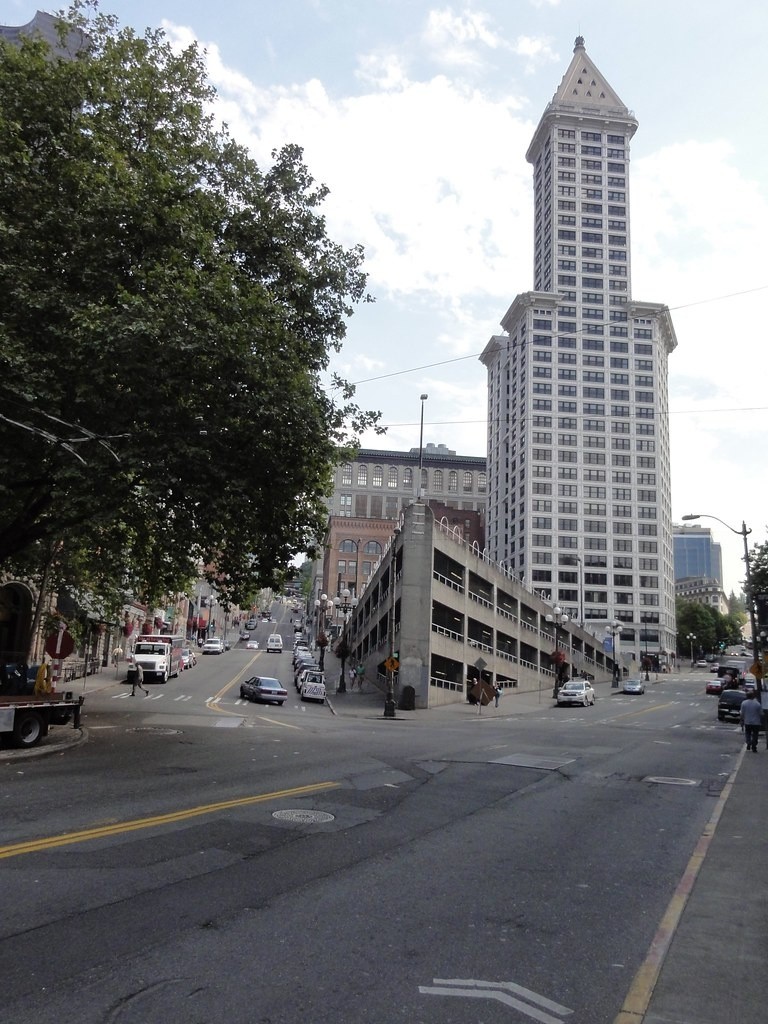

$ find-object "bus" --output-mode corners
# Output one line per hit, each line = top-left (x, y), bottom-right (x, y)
(127, 634), (184, 684)
(726, 661), (747, 684)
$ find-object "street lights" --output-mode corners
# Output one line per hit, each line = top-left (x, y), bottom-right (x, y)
(418, 393), (428, 501)
(334, 589), (358, 693)
(686, 633), (697, 668)
(756, 631), (768, 692)
(681, 512), (761, 689)
(313, 593), (334, 672)
(382, 527), (402, 717)
(605, 620), (622, 688)
(545, 607), (568, 699)
(204, 594), (218, 639)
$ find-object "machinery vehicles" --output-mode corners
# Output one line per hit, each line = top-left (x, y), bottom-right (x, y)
(0, 692), (86, 747)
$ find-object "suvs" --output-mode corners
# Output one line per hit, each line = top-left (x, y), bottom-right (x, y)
(717, 690), (747, 721)
(717, 666), (740, 689)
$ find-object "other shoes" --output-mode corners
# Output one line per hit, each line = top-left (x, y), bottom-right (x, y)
(146, 691), (149, 696)
(130, 694), (135, 697)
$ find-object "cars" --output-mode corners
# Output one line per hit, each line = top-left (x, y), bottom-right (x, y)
(730, 651), (738, 656)
(239, 675), (288, 706)
(291, 601), (302, 613)
(183, 649), (193, 669)
(246, 641), (260, 650)
(697, 660), (707, 667)
(740, 652), (753, 657)
(557, 680), (595, 707)
(202, 639), (230, 654)
(240, 631), (249, 641)
(623, 680), (645, 695)
(745, 678), (755, 690)
(710, 663), (720, 672)
(705, 679), (729, 694)
(246, 620), (258, 630)
(292, 620), (327, 704)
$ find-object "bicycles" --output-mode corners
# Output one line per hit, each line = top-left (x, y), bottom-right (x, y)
(193, 660), (197, 666)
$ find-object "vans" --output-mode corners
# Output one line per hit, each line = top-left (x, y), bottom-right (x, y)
(267, 634), (283, 653)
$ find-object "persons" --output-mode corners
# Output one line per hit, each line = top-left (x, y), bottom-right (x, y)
(190, 634), (196, 643)
(111, 645), (123, 666)
(740, 692), (764, 753)
(472, 678), (479, 706)
(493, 681), (501, 707)
(197, 637), (203, 648)
(349, 663), (365, 690)
(662, 663), (680, 673)
(129, 663), (149, 697)
